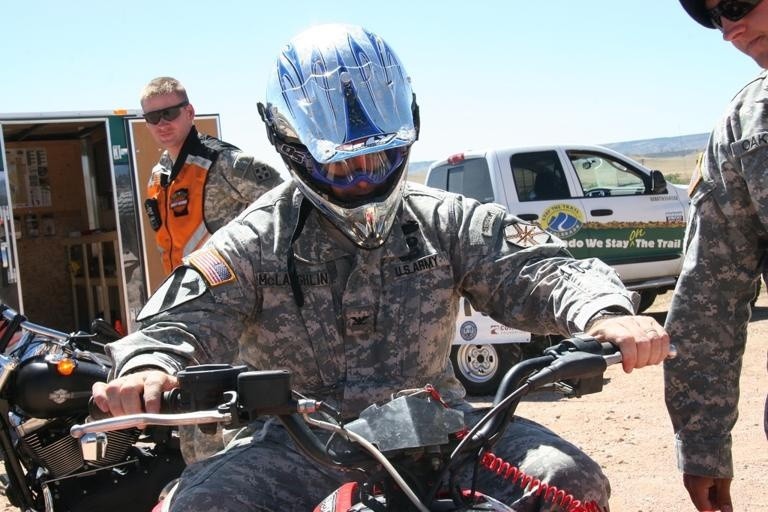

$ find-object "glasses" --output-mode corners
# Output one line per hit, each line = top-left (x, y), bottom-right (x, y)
(689, 0), (762, 30)
(141, 101), (190, 125)
(276, 141), (413, 189)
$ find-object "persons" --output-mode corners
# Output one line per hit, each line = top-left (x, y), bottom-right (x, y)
(141, 77), (285, 277)
(662, 1), (767, 511)
(92, 21), (670, 512)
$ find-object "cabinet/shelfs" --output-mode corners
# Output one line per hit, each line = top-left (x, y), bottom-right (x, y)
(65, 230), (126, 334)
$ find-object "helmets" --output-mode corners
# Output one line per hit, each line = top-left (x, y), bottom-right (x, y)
(257, 24), (420, 251)
(680, 0), (718, 30)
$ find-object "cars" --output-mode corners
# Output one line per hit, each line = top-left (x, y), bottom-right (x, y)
(425, 144), (761, 395)
